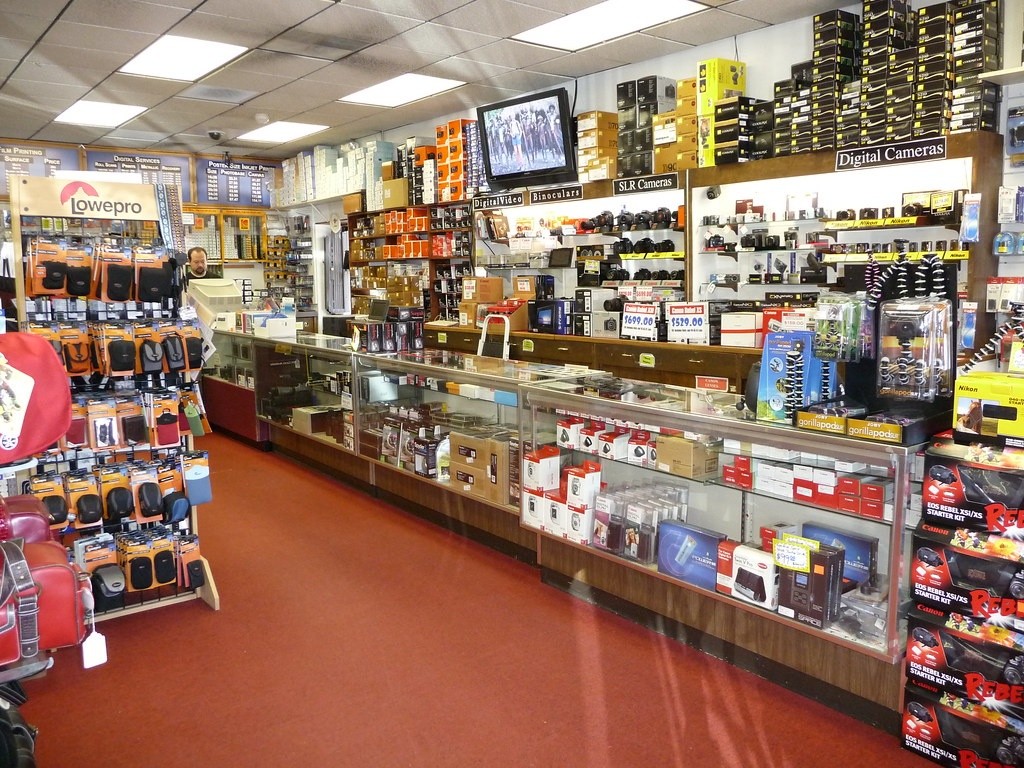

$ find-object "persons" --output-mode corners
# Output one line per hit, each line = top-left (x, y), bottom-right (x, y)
(181, 248), (222, 291)
(486, 104), (564, 172)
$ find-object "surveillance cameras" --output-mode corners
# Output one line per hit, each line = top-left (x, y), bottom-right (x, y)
(209, 132), (221, 141)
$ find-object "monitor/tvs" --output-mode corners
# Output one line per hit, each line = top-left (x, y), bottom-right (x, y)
(477, 86), (579, 192)
(537, 305), (555, 331)
(189, 278), (242, 325)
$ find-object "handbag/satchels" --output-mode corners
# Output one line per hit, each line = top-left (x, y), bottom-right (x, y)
(0, 666), (38, 768)
(0, 258), (16, 298)
(0, 332), (72, 472)
(0, 494), (86, 665)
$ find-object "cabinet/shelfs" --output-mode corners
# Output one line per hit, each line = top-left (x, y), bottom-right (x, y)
(1, 452), (52, 687)
(348, 205), (430, 318)
(475, 168), (688, 383)
(202, 328), (934, 714)
(425, 198), (474, 352)
(689, 132), (1003, 395)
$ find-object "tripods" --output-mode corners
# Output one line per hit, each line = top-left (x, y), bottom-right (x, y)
(915, 254), (947, 298)
(868, 239), (914, 307)
(879, 340), (928, 388)
(864, 248), (880, 292)
(960, 302), (1024, 376)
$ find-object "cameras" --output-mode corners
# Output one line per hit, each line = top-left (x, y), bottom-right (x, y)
(580, 208), (685, 311)
(884, 313), (928, 340)
(703, 185), (969, 286)
(604, 317), (616, 331)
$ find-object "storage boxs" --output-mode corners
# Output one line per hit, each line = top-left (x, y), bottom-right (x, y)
(232, 0), (1002, 354)
(846, 400), (953, 448)
(899, 371), (1024, 768)
(797, 390), (896, 436)
